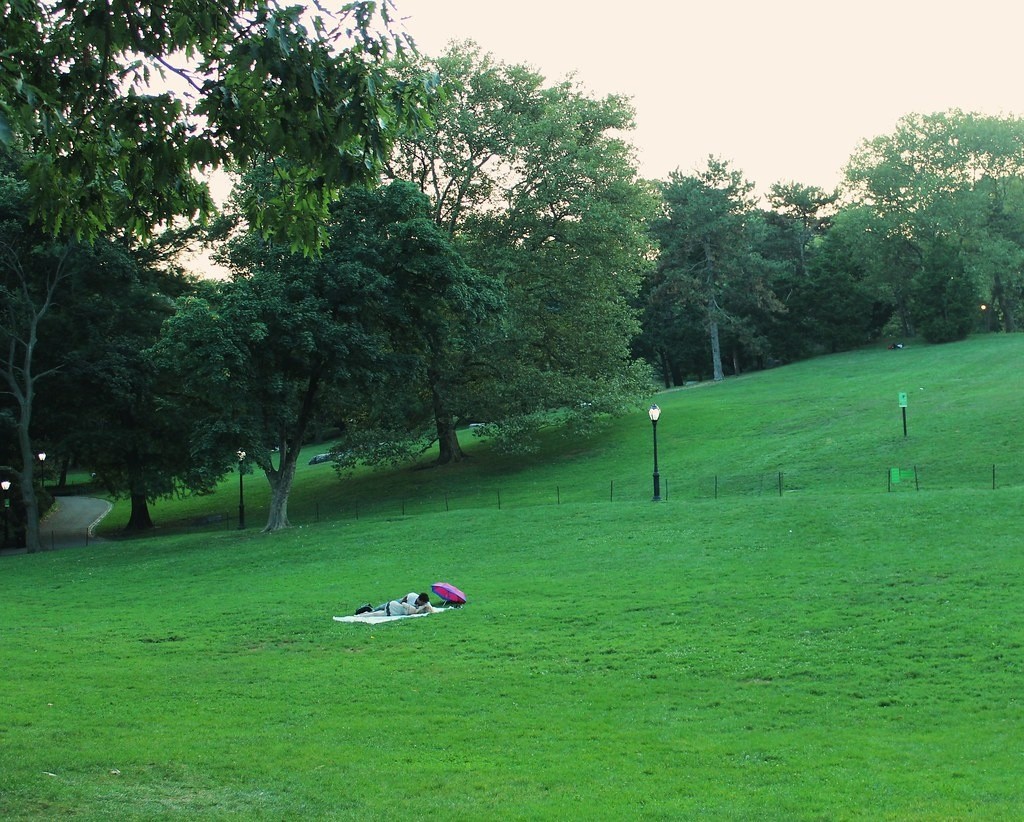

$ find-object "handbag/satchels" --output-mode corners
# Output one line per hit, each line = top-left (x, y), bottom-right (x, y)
(355, 604), (373, 615)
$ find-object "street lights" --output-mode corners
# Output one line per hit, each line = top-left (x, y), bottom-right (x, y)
(649, 403), (661, 501)
(0, 479), (12, 548)
(236, 450), (247, 530)
(37, 451), (47, 491)
(980, 304), (987, 332)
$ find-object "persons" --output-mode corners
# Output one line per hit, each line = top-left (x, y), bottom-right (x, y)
(892, 342), (904, 350)
(354, 592), (434, 616)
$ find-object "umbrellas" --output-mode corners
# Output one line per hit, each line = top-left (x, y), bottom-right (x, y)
(431, 582), (466, 607)
